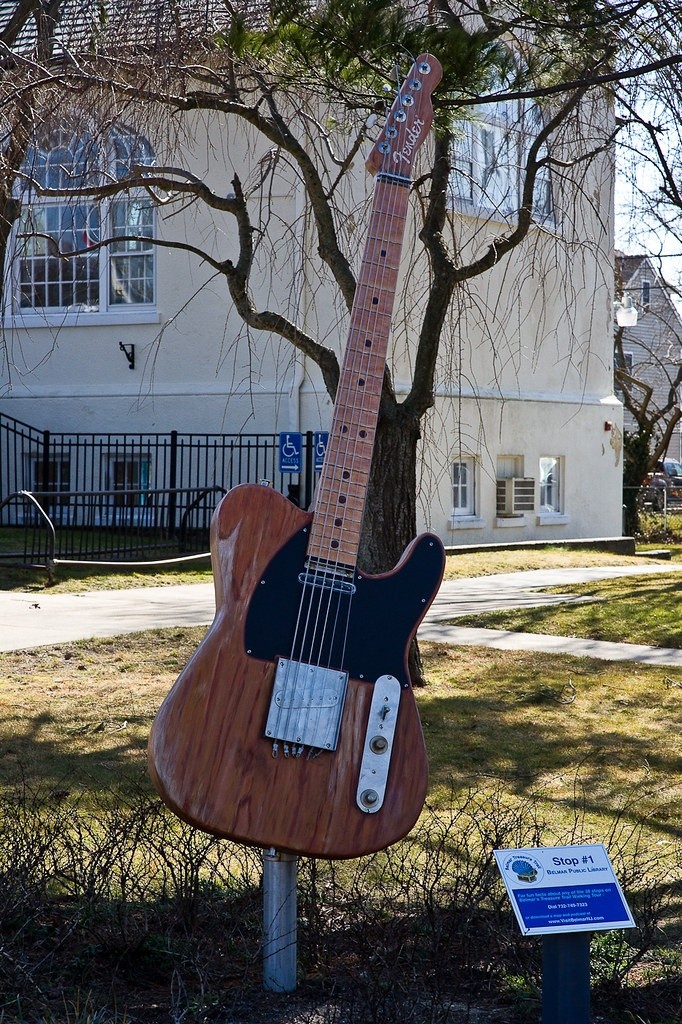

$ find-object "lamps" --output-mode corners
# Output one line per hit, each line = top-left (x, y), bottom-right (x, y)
(612, 297), (637, 327)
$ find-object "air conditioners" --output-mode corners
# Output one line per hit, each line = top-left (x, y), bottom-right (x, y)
(496, 478), (537, 516)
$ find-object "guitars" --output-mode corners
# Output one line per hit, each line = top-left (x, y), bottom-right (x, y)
(140, 48), (453, 863)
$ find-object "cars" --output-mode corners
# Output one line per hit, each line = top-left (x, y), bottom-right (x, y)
(623, 457), (682, 511)
